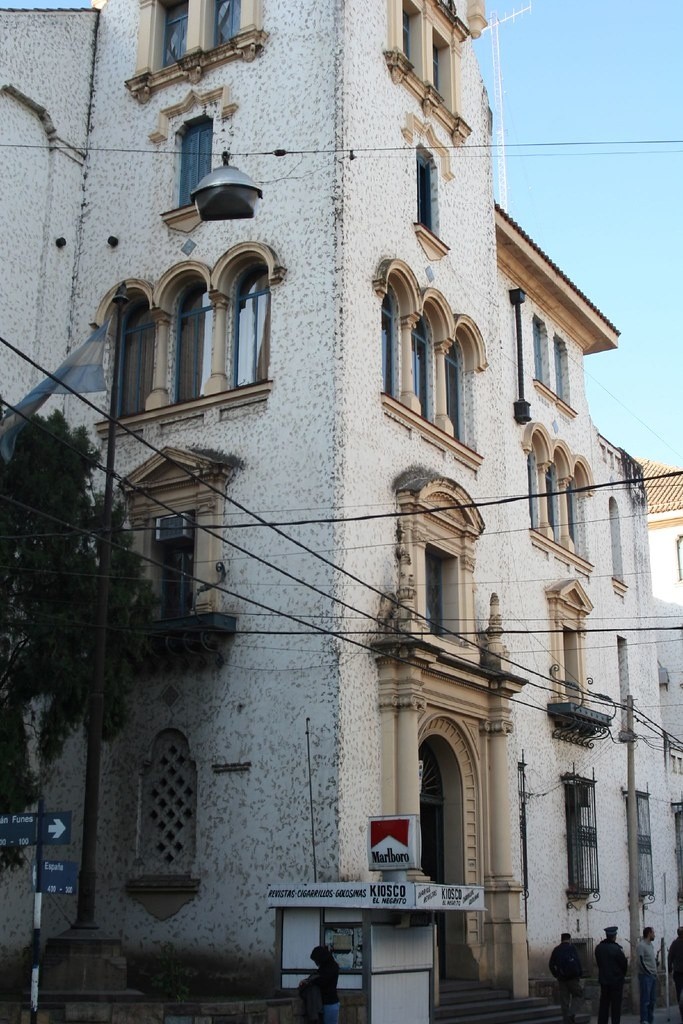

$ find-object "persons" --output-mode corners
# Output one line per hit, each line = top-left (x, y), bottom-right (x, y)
(668, 927), (683, 1018)
(311, 945), (341, 1024)
(595, 926), (628, 1024)
(549, 933), (584, 1024)
(635, 927), (659, 1024)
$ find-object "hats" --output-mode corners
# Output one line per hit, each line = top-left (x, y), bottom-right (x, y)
(312, 946), (328, 958)
(604, 927), (618, 936)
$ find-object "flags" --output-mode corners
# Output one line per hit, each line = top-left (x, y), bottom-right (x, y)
(0, 318), (112, 462)
